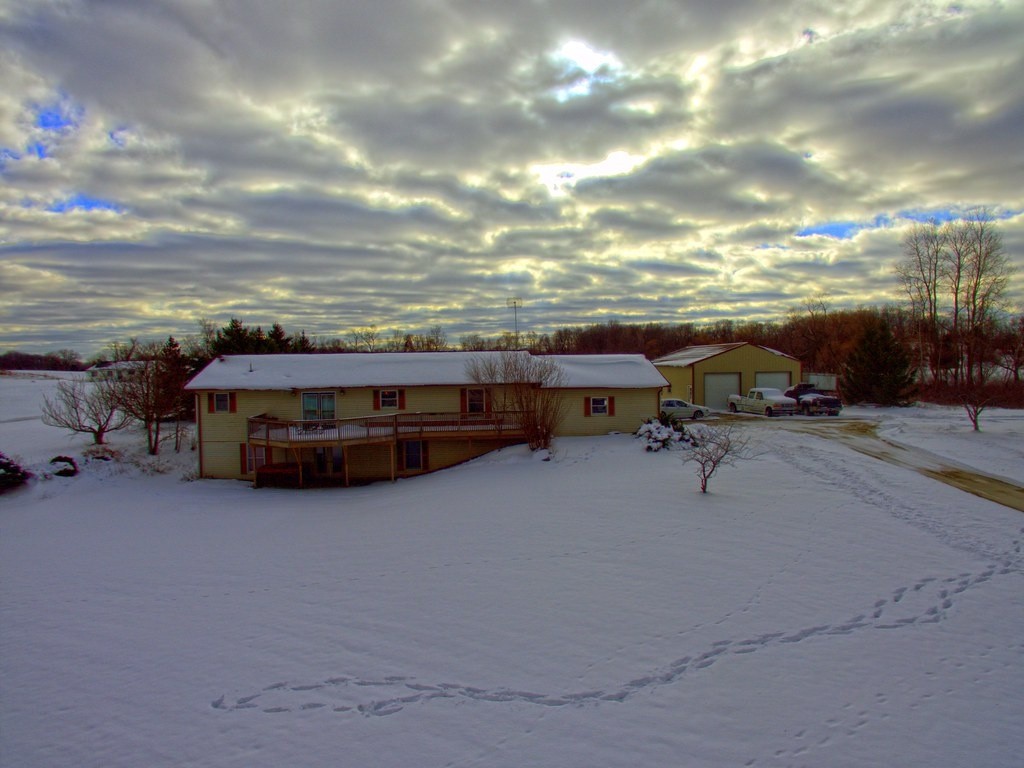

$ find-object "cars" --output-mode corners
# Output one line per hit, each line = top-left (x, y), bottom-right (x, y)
(660, 399), (710, 420)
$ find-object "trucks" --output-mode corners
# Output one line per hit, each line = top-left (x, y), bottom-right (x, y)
(782, 381), (842, 416)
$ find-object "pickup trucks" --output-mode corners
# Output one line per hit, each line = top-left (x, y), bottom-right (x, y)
(726, 387), (798, 417)
